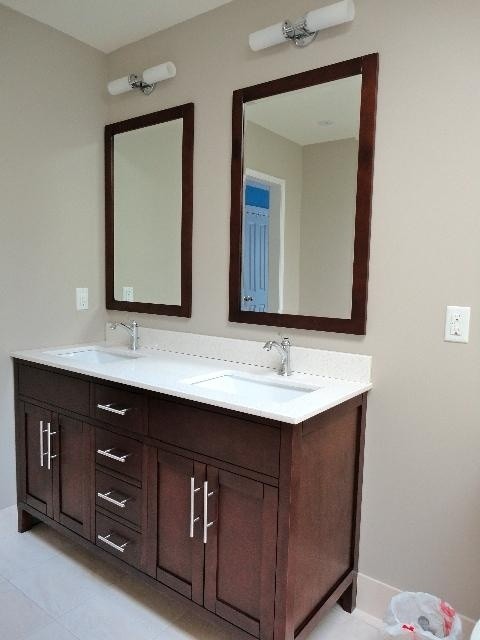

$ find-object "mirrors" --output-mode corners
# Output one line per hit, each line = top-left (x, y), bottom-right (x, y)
(103, 102), (195, 318)
(228, 53), (380, 336)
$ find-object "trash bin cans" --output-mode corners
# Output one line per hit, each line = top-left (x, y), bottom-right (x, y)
(391, 593), (462, 639)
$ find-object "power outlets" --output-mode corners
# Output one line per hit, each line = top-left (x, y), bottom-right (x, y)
(444, 305), (471, 344)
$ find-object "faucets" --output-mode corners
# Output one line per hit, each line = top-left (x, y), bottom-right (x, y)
(110, 319), (141, 350)
(262, 337), (293, 376)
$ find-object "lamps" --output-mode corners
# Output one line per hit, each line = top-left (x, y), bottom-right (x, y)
(107, 61), (178, 98)
(248, 0), (356, 53)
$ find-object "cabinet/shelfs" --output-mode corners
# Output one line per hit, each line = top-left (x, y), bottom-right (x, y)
(87, 376), (158, 591)
(146, 387), (368, 640)
(11, 355), (93, 549)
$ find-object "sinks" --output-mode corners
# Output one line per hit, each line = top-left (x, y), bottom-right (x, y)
(180, 369), (324, 419)
(42, 345), (144, 370)
